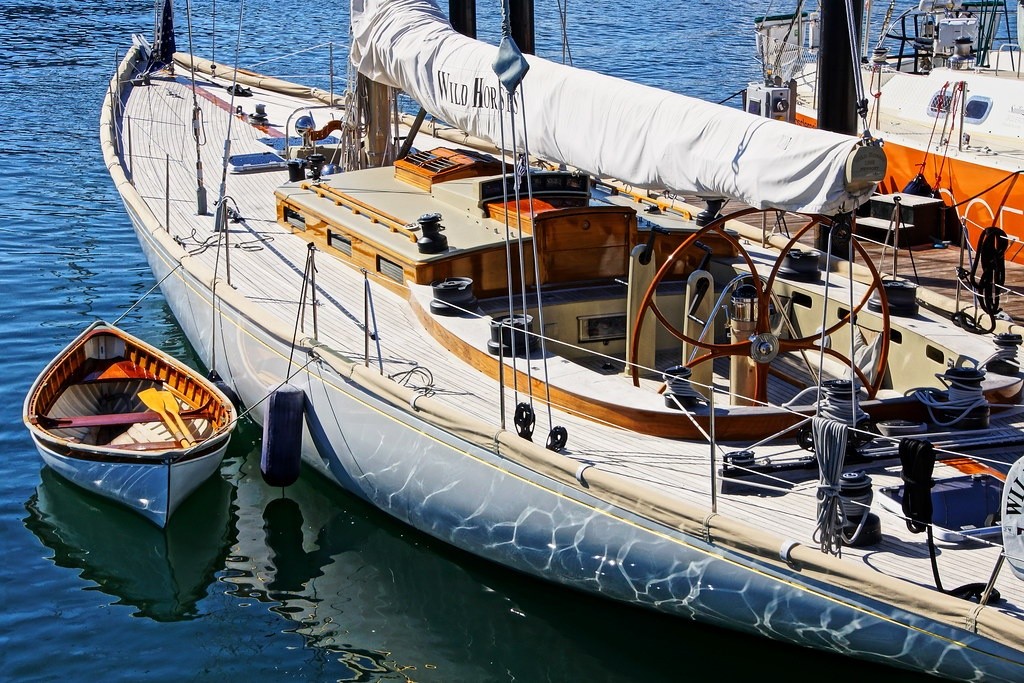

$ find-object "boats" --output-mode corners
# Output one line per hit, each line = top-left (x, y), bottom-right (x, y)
(25, 463), (239, 621)
(22, 320), (238, 529)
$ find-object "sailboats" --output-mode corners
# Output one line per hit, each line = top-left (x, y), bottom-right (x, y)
(100, 0), (1024, 683)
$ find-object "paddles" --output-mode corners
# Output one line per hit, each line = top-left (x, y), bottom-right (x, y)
(135, 387), (198, 449)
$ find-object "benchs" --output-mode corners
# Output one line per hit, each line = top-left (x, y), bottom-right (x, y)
(100, 438), (208, 451)
(44, 408), (217, 431)
